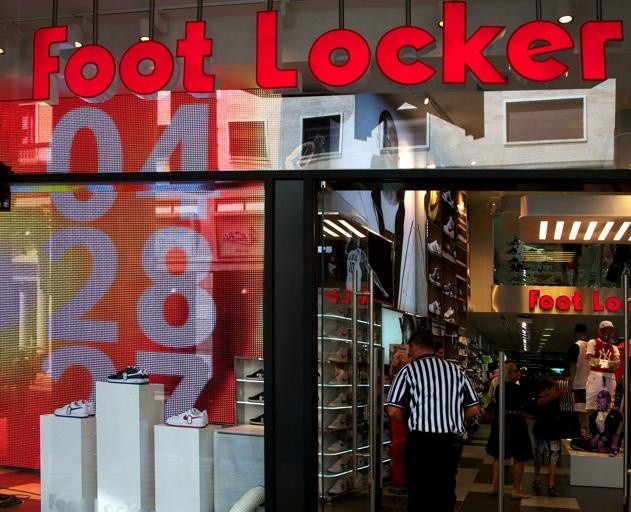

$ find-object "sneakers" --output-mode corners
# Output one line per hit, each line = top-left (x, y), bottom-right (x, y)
(107, 368), (149, 384)
(250, 414), (264, 425)
(428, 190), (456, 325)
(485, 480), (560, 499)
(248, 392), (264, 403)
(326, 308), (391, 494)
(247, 368), (264, 381)
(167, 408), (208, 428)
(55, 399), (96, 418)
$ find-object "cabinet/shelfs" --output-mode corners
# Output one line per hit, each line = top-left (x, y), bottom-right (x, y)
(234, 358), (265, 425)
(319, 286), (392, 499)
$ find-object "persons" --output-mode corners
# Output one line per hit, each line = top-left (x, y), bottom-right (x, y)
(586, 321), (621, 440)
(570, 389), (622, 457)
(570, 322), (590, 439)
(489, 359), (568, 497)
(434, 338), (444, 359)
(383, 330), (481, 512)
(14, 350), (37, 413)
(286, 135), (327, 168)
(369, 109), (402, 232)
(345, 235), (389, 299)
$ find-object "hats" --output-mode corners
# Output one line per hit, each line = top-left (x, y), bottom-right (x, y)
(600, 320), (614, 330)
